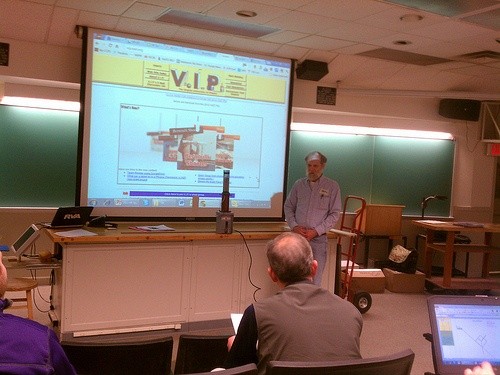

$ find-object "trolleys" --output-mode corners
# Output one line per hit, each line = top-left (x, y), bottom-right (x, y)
(335, 196), (373, 315)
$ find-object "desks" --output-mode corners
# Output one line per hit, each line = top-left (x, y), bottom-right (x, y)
(39, 219), (340, 343)
(1, 255), (62, 270)
(410, 219), (500, 291)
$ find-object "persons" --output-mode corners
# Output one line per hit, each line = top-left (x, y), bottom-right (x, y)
(284, 151), (341, 287)
(224, 231), (364, 370)
(465, 361), (495, 375)
(0, 250), (79, 375)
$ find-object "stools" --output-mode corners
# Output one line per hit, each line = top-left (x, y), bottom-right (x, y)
(4, 278), (39, 320)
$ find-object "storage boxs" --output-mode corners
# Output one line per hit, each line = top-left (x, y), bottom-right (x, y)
(340, 267), (386, 294)
(383, 267), (426, 293)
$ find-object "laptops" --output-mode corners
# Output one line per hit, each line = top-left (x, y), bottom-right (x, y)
(427, 294), (500, 375)
(40, 206), (94, 228)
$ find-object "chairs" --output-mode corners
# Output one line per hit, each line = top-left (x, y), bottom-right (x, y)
(174, 334), (229, 375)
(187, 363), (258, 375)
(267, 347), (415, 375)
(58, 336), (174, 375)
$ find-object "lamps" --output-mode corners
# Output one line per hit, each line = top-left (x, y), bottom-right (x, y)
(290, 121), (456, 144)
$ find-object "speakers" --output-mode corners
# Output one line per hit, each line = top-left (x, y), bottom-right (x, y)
(296, 59), (329, 81)
(438, 99), (481, 121)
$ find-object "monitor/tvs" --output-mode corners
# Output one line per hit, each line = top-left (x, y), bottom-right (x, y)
(11, 224), (39, 256)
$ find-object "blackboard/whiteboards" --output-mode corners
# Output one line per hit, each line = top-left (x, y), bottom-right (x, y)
(0, 102), (80, 210)
(285, 129), (457, 219)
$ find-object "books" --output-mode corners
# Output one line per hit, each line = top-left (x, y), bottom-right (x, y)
(453, 223), (483, 228)
